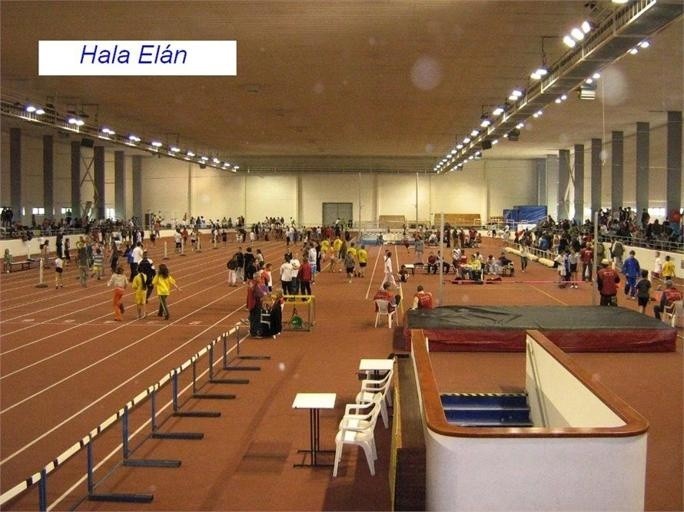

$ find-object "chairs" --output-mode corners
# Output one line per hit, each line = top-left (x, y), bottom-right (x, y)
(372, 297), (399, 330)
(662, 300), (683, 328)
(329, 356), (395, 481)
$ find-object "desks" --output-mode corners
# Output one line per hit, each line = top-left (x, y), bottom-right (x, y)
(289, 392), (339, 469)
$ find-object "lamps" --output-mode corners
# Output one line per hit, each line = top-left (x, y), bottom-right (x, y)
(431, 0), (684, 176)
(0, 96), (240, 174)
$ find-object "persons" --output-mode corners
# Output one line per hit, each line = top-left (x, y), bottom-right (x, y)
(0, 205), (684, 339)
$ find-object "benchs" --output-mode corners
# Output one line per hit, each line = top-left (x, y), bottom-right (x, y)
(504, 246), (555, 269)
(4, 254), (75, 271)
(404, 262), (512, 277)
